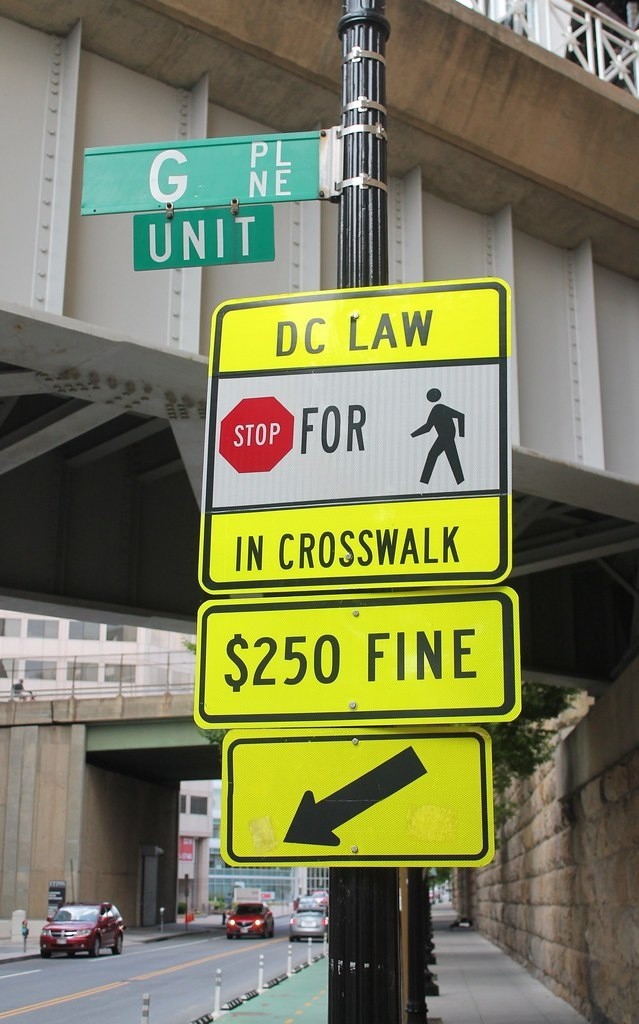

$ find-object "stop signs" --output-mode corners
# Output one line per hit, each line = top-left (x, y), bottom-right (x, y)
(197, 278), (517, 599)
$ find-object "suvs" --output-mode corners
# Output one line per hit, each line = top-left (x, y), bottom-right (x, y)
(40, 901), (125, 959)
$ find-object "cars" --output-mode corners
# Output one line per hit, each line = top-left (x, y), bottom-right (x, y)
(289, 891), (329, 943)
(226, 902), (275, 940)
